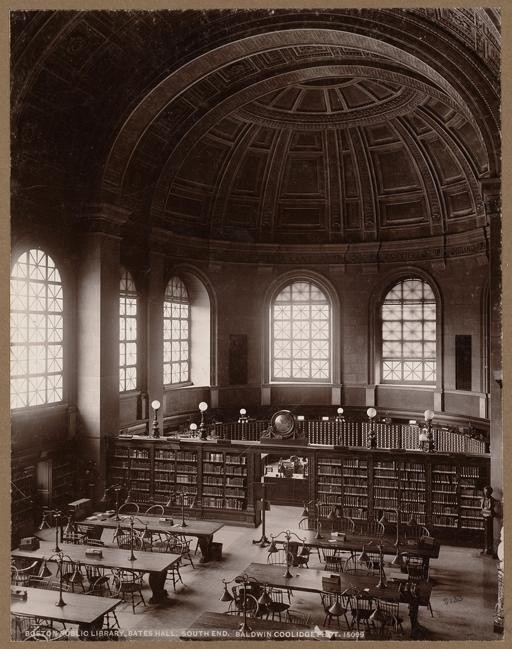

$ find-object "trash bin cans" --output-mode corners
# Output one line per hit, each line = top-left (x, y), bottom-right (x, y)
(209, 542), (223, 560)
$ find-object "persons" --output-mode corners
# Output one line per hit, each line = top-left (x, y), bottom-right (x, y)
(480, 487), (496, 519)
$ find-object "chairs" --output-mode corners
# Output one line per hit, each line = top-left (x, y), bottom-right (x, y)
(11, 501), (445, 641)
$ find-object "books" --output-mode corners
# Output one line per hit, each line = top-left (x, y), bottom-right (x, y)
(109, 446), (247, 511)
(316, 457), (487, 529)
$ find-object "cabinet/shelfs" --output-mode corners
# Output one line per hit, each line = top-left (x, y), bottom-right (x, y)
(109, 434), (263, 528)
(37, 448), (76, 520)
(265, 471), (307, 507)
(12, 470), (35, 551)
(308, 445), (491, 549)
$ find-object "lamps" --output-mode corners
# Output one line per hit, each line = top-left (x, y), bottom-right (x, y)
(145, 398), (439, 452)
